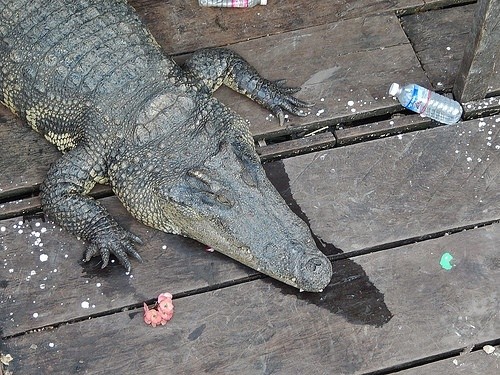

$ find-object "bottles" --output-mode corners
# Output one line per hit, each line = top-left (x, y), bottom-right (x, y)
(389, 83), (462, 125)
(198, 0), (268, 8)
